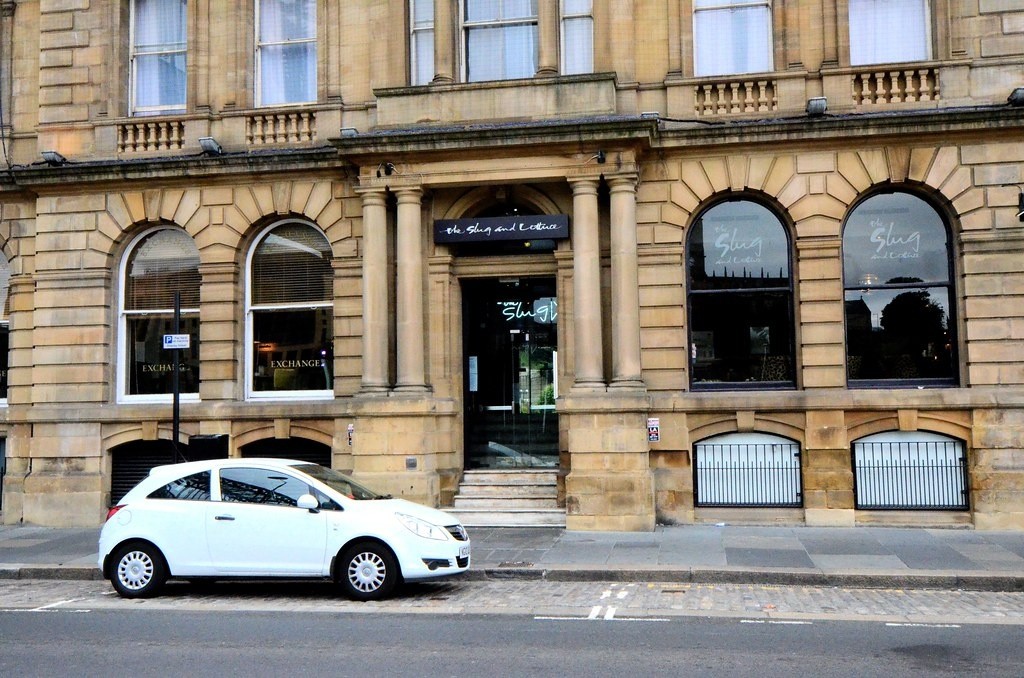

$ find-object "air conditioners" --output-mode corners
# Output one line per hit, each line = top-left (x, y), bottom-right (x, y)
(198, 137), (222, 156)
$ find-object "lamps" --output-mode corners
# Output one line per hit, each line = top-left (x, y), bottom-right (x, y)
(804, 95), (828, 115)
(1007, 88), (1024, 107)
(41, 150), (67, 168)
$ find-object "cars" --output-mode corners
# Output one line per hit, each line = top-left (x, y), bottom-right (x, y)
(467, 441), (545, 470)
(92, 458), (473, 603)
